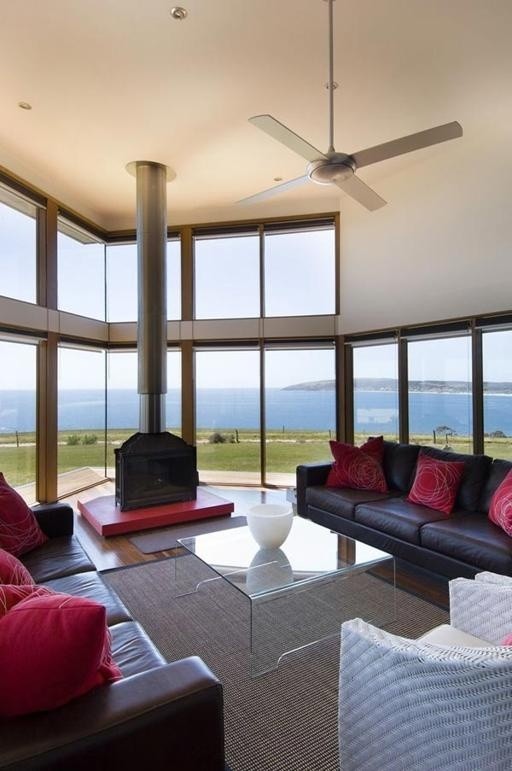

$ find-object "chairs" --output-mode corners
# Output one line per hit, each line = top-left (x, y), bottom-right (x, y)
(336, 571), (511, 771)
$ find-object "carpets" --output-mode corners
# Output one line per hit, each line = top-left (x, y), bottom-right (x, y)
(103, 544), (450, 771)
(127, 516), (248, 555)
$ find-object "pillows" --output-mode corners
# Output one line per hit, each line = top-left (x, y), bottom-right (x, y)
(0, 470), (124, 722)
(326, 435), (511, 538)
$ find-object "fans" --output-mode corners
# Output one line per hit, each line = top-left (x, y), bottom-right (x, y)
(232, 0), (465, 213)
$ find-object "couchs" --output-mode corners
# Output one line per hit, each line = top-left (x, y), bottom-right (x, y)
(296, 437), (512, 587)
(0, 501), (228, 771)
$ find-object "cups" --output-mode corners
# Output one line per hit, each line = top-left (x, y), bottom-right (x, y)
(245, 502), (294, 549)
